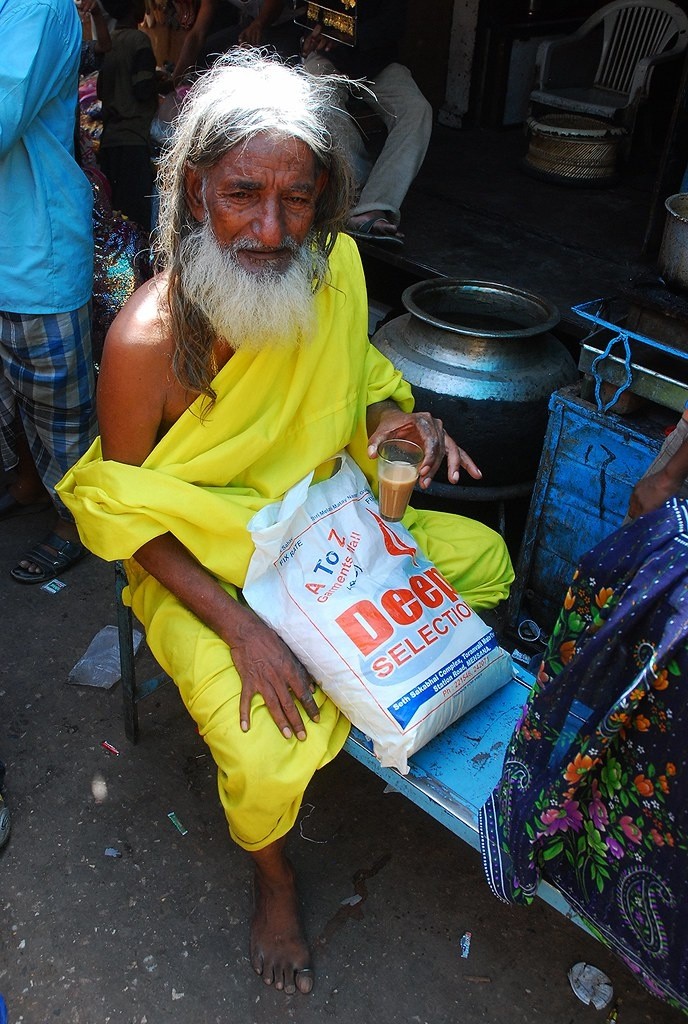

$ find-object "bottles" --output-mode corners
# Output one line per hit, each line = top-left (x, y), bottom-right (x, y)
(370, 277), (579, 490)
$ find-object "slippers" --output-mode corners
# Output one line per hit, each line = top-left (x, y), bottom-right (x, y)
(0, 483), (52, 521)
(10, 533), (90, 583)
(341, 218), (404, 245)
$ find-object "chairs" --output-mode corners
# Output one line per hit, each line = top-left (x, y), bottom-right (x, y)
(522, 0), (688, 171)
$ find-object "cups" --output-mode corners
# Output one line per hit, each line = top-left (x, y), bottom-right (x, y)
(376, 439), (424, 522)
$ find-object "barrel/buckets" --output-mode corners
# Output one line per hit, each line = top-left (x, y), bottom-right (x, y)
(658, 193), (688, 285)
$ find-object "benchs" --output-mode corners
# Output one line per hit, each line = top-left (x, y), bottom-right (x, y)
(111, 554), (610, 953)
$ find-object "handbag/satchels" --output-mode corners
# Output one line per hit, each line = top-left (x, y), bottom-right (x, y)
(242, 450), (513, 776)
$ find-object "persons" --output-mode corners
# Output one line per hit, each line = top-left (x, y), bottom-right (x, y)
(75, 0), (434, 246)
(623, 410), (687, 528)
(0, 0), (95, 583)
(53, 44), (483, 992)
